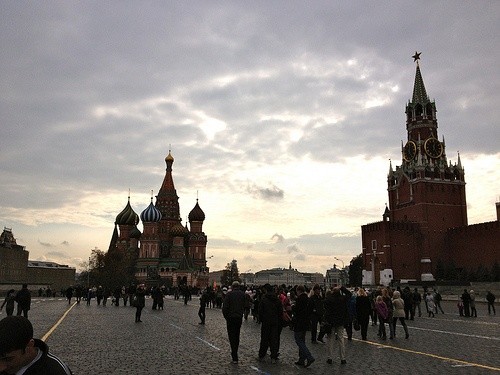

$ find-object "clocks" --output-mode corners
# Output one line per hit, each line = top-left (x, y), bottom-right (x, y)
(424, 138), (443, 158)
(403, 141), (416, 162)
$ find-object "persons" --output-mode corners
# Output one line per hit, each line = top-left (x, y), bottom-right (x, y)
(207, 283), (415, 340)
(486, 291), (496, 315)
(16, 283), (31, 318)
(257, 285), (283, 360)
(223, 281), (250, 362)
(412, 287), (444, 318)
(0, 315), (72, 375)
(457, 288), (477, 318)
(0, 289), (16, 316)
(135, 285), (145, 322)
(38, 288), (63, 297)
(66, 284), (207, 311)
(323, 284), (353, 364)
(289, 286), (316, 368)
(198, 290), (207, 324)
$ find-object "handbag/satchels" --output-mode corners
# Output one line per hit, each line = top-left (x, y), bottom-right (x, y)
(131, 299), (139, 307)
(282, 307), (292, 323)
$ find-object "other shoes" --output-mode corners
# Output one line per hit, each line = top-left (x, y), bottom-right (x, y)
(394, 334), (396, 336)
(312, 340), (318, 344)
(383, 336), (387, 340)
(327, 359), (332, 364)
(270, 354), (277, 358)
(199, 322), (204, 324)
(135, 320), (142, 322)
(294, 360), (304, 367)
(232, 359), (238, 363)
(317, 338), (326, 343)
(256, 357), (265, 362)
(389, 337), (393, 340)
(304, 357), (315, 368)
(405, 334), (409, 338)
(341, 360), (346, 364)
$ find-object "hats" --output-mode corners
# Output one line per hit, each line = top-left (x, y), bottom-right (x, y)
(470, 290), (474, 293)
